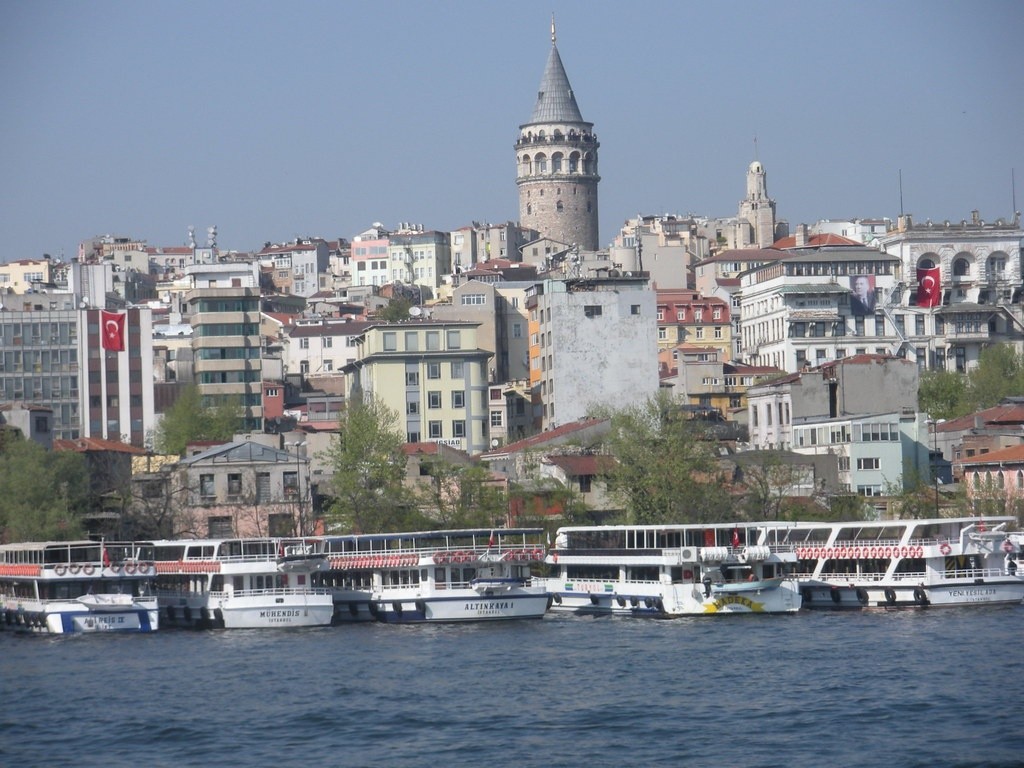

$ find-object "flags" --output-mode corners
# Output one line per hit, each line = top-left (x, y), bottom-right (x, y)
(733, 528), (739, 547)
(489, 535), (495, 548)
(101, 309), (126, 353)
(979, 521), (984, 532)
(103, 545), (109, 567)
(916, 267), (940, 306)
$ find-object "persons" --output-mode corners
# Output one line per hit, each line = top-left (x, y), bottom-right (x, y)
(851, 276), (876, 315)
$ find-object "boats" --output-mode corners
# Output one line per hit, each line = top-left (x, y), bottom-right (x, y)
(754, 515), (1024, 607)
(539, 524), (803, 618)
(146, 538), (335, 630)
(317, 526), (552, 624)
(0, 539), (160, 635)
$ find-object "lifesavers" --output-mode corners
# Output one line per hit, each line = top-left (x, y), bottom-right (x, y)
(553, 554), (558, 562)
(349, 599), (425, 615)
(502, 549), (543, 560)
(0, 565), (41, 576)
(166, 605), (222, 623)
(940, 543), (952, 555)
(153, 562), (222, 573)
(855, 546), (923, 558)
(914, 587), (926, 604)
(794, 546), (833, 559)
(55, 562), (150, 577)
(834, 548), (840, 558)
(802, 587), (896, 602)
(841, 548), (846, 557)
(433, 551), (476, 564)
(848, 547), (853, 559)
(4, 610), (47, 627)
(1004, 542), (1013, 552)
(553, 593), (661, 609)
(328, 554), (420, 568)
